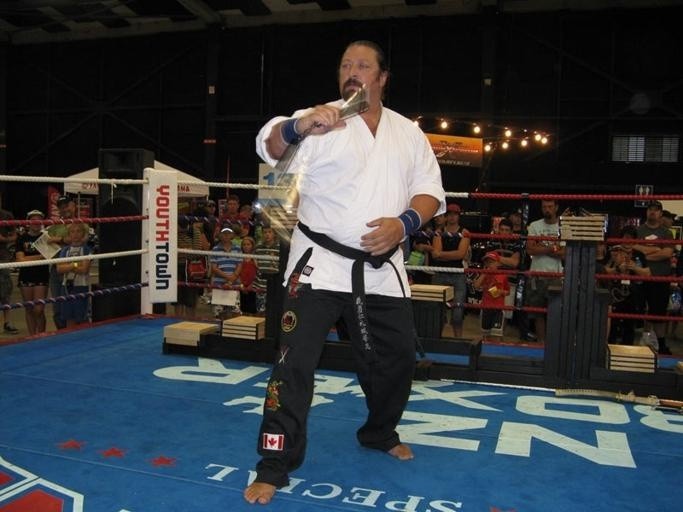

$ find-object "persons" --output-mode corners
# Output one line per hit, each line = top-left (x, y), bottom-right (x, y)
(0, 195), (100, 337)
(595, 199), (683, 357)
(400, 198), (565, 356)
(243, 40), (448, 505)
(172, 195), (299, 315)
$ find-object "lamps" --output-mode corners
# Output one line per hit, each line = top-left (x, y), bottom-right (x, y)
(413, 115), (553, 152)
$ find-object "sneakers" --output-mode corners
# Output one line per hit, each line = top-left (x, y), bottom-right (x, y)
(3, 322), (19, 333)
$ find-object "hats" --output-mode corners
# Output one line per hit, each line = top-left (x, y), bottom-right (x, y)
(482, 251), (500, 262)
(220, 224), (233, 233)
(447, 203), (461, 212)
(27, 210), (45, 220)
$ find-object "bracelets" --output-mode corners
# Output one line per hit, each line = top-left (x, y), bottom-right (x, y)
(279, 118), (303, 146)
